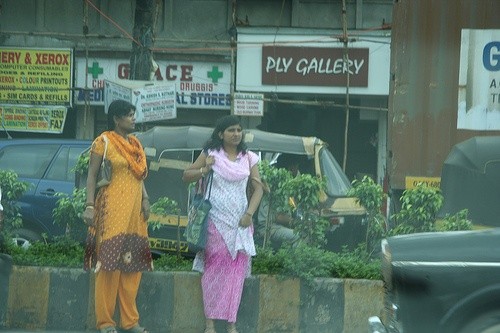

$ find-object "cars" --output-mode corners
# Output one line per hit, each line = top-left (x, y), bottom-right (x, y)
(368, 227), (500, 333)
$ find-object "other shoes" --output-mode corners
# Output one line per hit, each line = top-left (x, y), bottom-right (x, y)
(100, 326), (117, 333)
(120, 326), (148, 333)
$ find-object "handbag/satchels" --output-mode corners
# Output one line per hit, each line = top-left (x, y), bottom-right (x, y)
(75, 135), (111, 192)
(182, 150), (214, 249)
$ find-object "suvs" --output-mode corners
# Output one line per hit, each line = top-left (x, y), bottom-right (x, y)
(0, 139), (93, 255)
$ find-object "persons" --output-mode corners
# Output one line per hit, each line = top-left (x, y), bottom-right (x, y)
(258, 153), (306, 249)
(182, 114), (263, 333)
(80, 99), (152, 333)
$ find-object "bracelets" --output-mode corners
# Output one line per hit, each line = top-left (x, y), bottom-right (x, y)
(244, 212), (253, 217)
(84, 202), (95, 209)
(142, 196), (151, 202)
(200, 168), (207, 179)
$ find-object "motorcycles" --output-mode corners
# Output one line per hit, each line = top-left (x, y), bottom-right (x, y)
(126, 125), (368, 261)
(439, 136), (500, 227)
